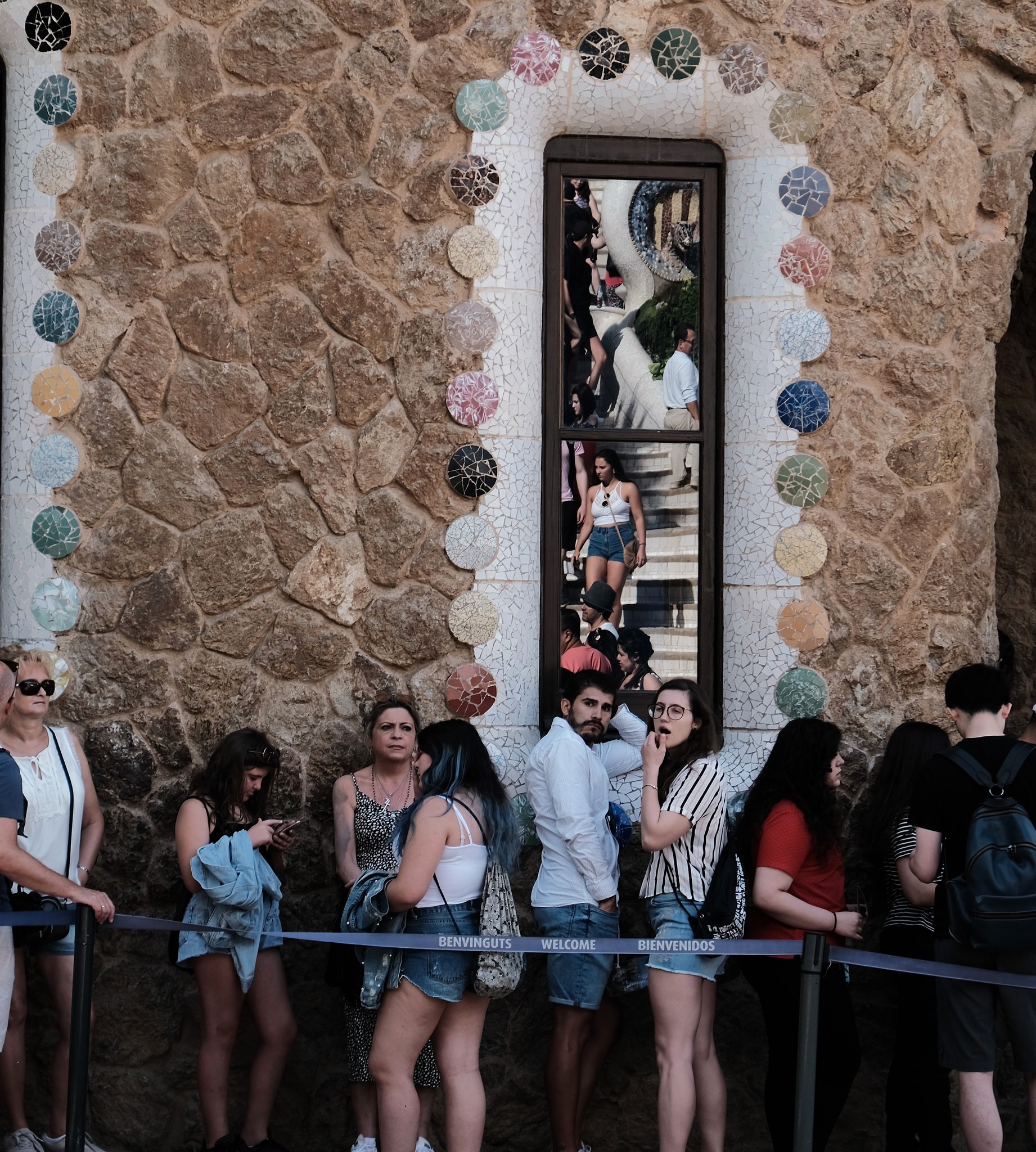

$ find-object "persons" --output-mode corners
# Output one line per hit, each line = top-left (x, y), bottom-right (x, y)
(557, 174), (699, 696)
(0, 648), (1036, 1152)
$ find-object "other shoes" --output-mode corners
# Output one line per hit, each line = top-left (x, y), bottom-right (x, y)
(670, 478), (686, 488)
(560, 582), (570, 607)
(200, 1132), (290, 1152)
(593, 410), (604, 423)
(414, 1136), (434, 1152)
(350, 1133), (377, 1152)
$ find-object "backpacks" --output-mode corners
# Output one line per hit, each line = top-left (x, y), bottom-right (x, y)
(433, 793), (525, 1000)
(660, 756), (747, 960)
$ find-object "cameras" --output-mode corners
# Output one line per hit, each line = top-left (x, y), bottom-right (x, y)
(11, 892), (71, 945)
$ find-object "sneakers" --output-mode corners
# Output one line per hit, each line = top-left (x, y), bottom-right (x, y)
(3, 1128), (45, 1152)
(41, 1129), (106, 1152)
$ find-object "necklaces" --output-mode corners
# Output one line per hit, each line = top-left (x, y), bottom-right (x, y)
(374, 769), (409, 804)
(379, 777), (406, 812)
(377, 771), (409, 812)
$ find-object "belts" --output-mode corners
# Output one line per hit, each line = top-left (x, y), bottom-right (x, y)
(668, 407), (688, 410)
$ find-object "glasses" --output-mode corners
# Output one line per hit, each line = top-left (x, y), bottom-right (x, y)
(594, 627), (602, 641)
(15, 679), (55, 696)
(369, 692), (416, 711)
(248, 747), (281, 762)
(646, 704), (699, 720)
(0, 659), (19, 703)
(856, 885), (868, 928)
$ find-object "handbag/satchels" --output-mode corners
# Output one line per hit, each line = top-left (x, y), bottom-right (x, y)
(623, 538), (639, 569)
(9, 891), (44, 949)
(941, 740), (1036, 969)
(40, 896), (69, 944)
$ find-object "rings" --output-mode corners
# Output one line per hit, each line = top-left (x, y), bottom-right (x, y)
(101, 905), (106, 908)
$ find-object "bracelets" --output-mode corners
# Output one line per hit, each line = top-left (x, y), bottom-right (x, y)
(602, 895), (615, 903)
(832, 912), (837, 932)
(77, 865), (90, 875)
(639, 543), (646, 546)
(641, 784), (658, 791)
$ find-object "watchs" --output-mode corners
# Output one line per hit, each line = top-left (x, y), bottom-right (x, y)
(569, 312), (575, 320)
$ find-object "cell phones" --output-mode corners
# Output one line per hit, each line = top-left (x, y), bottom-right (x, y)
(281, 821), (301, 833)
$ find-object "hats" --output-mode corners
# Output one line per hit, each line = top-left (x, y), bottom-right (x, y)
(578, 581), (616, 612)
